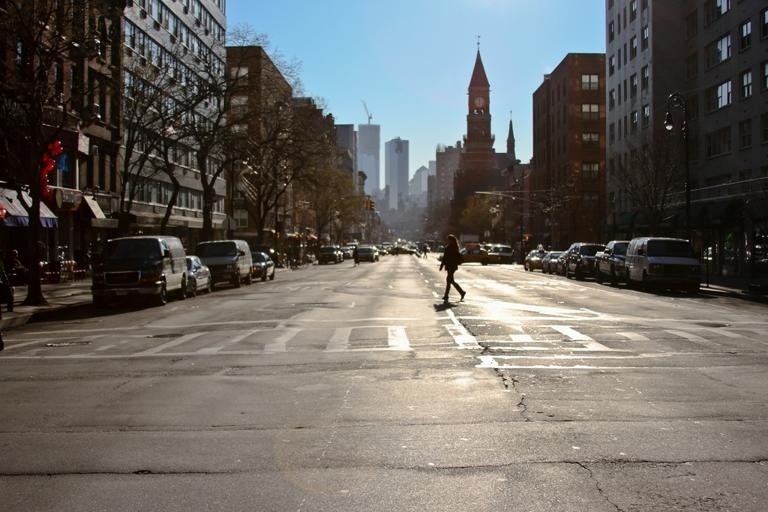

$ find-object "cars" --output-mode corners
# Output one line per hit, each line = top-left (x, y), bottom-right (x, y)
(438, 243), (516, 265)
(524, 249), (568, 276)
(251, 251), (275, 281)
(593, 240), (631, 286)
(186, 255), (212, 296)
(317, 238), (445, 265)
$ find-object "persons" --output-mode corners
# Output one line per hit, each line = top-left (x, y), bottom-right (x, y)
(351, 244), (361, 268)
(438, 233), (465, 302)
(421, 240), (428, 259)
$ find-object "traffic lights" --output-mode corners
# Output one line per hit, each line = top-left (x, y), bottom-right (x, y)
(364, 199), (375, 211)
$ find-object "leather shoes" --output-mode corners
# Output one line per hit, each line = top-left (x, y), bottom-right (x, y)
(460, 291), (466, 300)
(442, 296), (448, 300)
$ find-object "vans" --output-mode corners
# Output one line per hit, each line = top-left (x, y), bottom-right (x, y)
(196, 239), (254, 288)
(625, 236), (701, 294)
(90, 236), (189, 305)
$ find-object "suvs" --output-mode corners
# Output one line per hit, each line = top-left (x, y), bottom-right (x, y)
(566, 243), (606, 280)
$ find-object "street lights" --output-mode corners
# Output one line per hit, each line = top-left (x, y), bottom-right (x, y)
(230, 153), (259, 240)
(329, 197), (344, 245)
(496, 178), (523, 264)
(664, 91), (691, 240)
